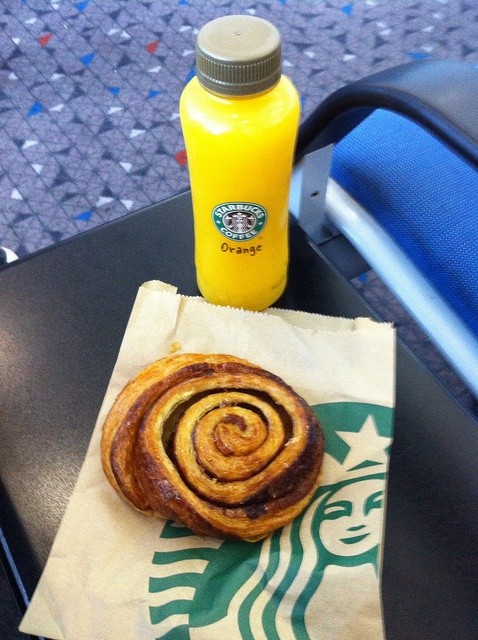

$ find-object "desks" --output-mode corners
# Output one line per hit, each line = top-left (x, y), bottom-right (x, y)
(0, 190), (477, 640)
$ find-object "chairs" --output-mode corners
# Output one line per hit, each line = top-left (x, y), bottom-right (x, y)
(294, 60), (477, 396)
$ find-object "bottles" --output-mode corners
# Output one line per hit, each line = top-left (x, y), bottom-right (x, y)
(180, 14), (301, 312)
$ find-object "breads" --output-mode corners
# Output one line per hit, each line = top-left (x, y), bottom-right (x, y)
(99, 351), (326, 543)
